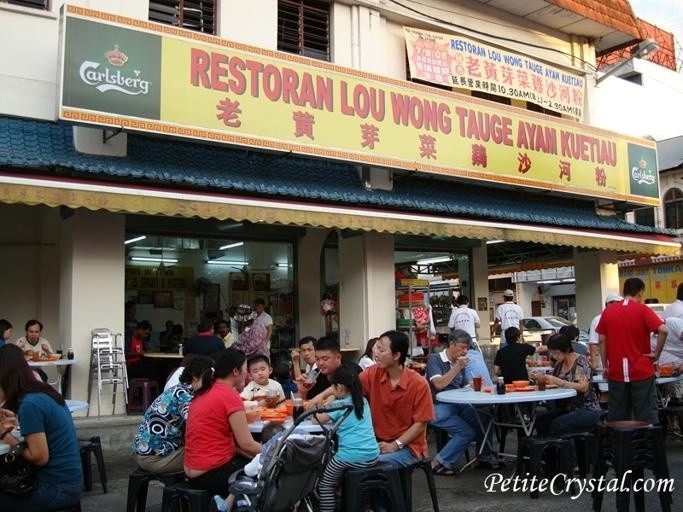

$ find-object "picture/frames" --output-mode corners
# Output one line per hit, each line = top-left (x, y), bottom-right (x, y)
(203, 284), (220, 310)
(153, 291), (173, 308)
(137, 290), (153, 304)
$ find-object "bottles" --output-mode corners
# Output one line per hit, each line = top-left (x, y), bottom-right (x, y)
(66, 348), (74, 360)
(419, 364), (425, 376)
(177, 343), (183, 355)
(496, 377), (504, 395)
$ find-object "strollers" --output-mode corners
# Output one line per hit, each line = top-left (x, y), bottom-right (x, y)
(224, 402), (354, 512)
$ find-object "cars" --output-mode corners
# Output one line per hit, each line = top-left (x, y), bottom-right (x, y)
(644, 303), (672, 317)
(502, 316), (591, 356)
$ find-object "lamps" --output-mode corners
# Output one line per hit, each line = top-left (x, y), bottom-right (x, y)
(218, 240), (246, 251)
(536, 284), (553, 295)
(596, 38), (659, 86)
(204, 259), (250, 266)
(123, 234), (148, 245)
(276, 262), (288, 267)
(129, 256), (181, 263)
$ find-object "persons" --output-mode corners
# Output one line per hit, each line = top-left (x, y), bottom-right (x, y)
(0, 277), (683, 512)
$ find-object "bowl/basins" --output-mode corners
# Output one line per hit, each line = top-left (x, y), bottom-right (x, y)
(526, 361), (538, 367)
(511, 380), (529, 387)
(542, 361), (550, 366)
(245, 410), (260, 424)
(48, 354), (60, 361)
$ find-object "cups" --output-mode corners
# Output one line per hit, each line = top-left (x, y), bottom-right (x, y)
(31, 348), (40, 362)
(55, 350), (62, 359)
(19, 339), (24, 350)
(472, 376), (481, 391)
(290, 398), (303, 424)
(285, 404), (293, 415)
(302, 369), (318, 390)
(537, 368), (546, 391)
(266, 390), (276, 408)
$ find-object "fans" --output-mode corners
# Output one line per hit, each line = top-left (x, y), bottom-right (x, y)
(232, 240), (275, 274)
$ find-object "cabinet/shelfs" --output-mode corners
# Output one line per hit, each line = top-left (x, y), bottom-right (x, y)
(268, 293), (295, 378)
(419, 286), (460, 327)
(229, 271), (270, 309)
(396, 286), (430, 374)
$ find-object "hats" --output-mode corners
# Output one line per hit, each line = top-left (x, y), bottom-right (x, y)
(502, 290), (514, 296)
(234, 304), (257, 322)
(606, 294), (624, 303)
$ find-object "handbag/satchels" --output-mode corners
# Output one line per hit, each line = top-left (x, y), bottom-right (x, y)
(0, 442), (35, 496)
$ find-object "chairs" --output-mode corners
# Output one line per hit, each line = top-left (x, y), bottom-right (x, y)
(85, 328), (129, 421)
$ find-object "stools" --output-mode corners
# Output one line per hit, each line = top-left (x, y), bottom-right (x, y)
(126, 469), (219, 512)
(526, 393), (683, 512)
(338, 455), (439, 512)
(127, 379), (159, 414)
(78, 436), (108, 493)
(428, 425), (471, 469)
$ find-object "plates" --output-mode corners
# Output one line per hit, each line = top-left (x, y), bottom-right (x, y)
(535, 384), (557, 389)
(261, 408), (286, 423)
(505, 385), (535, 391)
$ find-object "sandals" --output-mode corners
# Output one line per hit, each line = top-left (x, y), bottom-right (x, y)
(425, 460), (454, 476)
